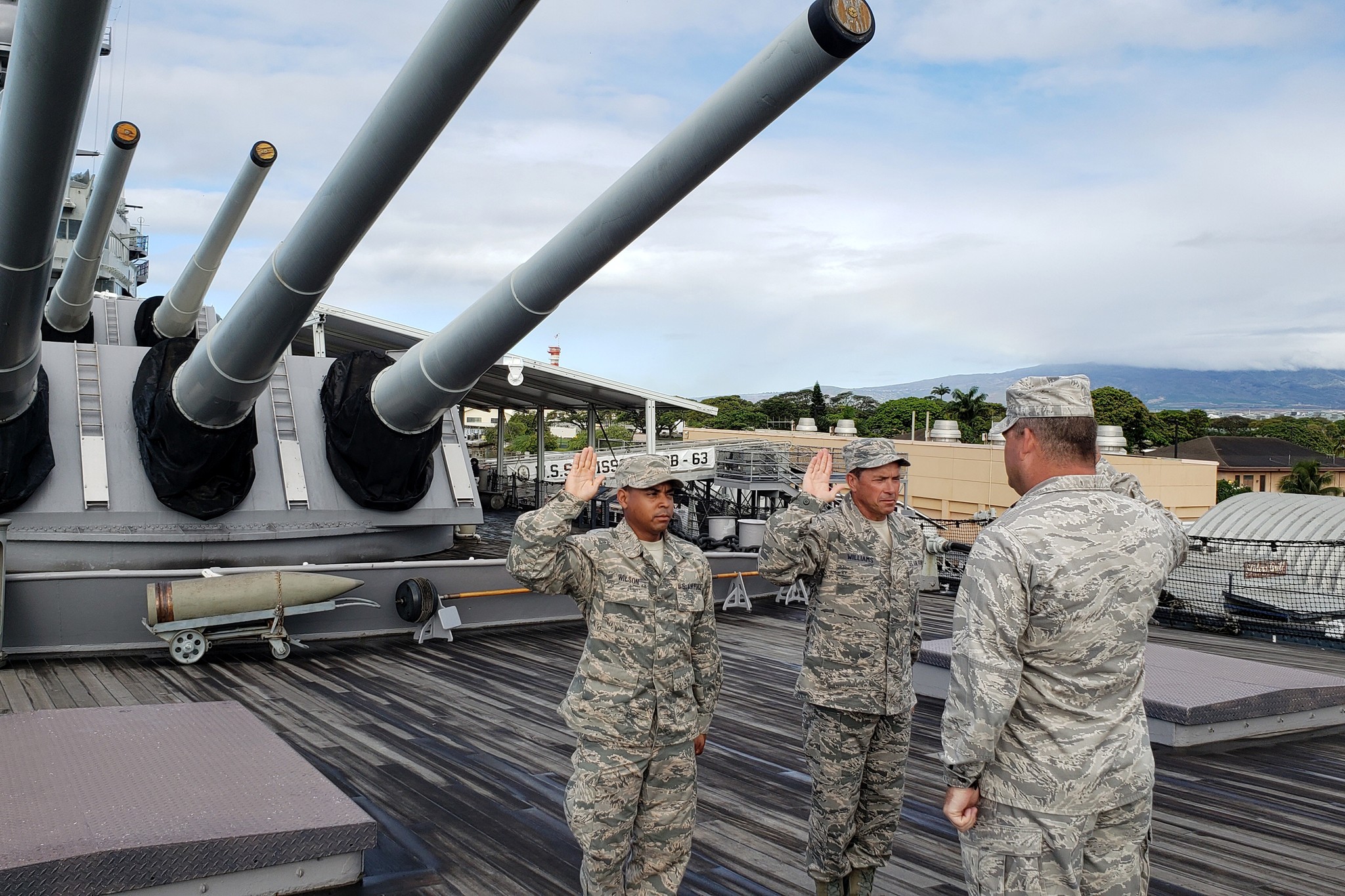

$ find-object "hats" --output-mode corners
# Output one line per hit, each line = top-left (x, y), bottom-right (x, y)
(989, 374), (1094, 434)
(615, 454), (684, 489)
(843, 438), (911, 474)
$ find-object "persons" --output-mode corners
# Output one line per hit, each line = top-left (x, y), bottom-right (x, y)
(756, 437), (927, 896)
(937, 374), (1190, 896)
(506, 446), (723, 896)
(470, 458), (480, 487)
